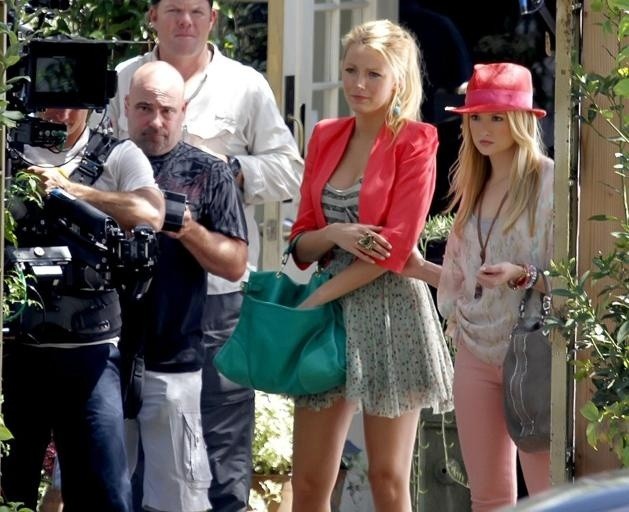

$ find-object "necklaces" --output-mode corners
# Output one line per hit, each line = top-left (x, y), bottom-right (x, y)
(473, 176), (512, 301)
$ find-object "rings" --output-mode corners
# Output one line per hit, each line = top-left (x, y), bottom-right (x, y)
(359, 231), (377, 251)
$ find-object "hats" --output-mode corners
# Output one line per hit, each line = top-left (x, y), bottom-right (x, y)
(444, 63), (547, 118)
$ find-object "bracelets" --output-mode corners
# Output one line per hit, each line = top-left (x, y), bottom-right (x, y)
(519, 264), (538, 290)
(508, 264), (529, 291)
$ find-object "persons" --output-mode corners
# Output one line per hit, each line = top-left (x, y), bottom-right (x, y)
(86, 0), (309, 512)
(1, 44), (168, 511)
(75, 61), (251, 512)
(398, 62), (553, 512)
(278, 18), (457, 512)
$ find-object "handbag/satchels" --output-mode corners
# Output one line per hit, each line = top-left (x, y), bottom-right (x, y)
(502, 264), (573, 453)
(213, 229), (346, 397)
